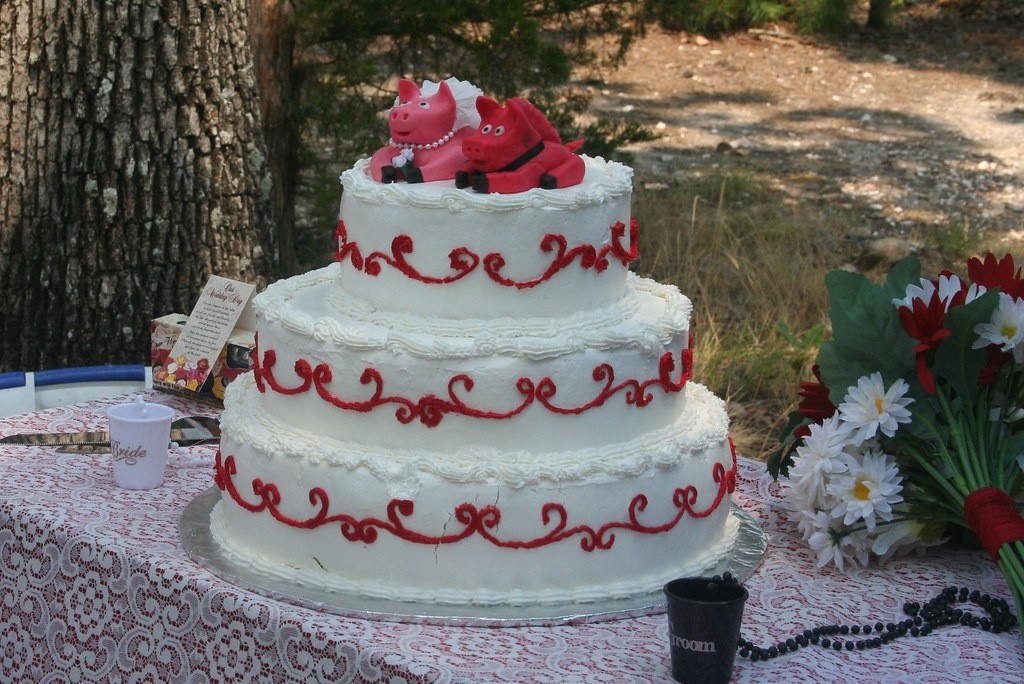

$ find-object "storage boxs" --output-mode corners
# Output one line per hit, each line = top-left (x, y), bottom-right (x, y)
(148, 313), (258, 407)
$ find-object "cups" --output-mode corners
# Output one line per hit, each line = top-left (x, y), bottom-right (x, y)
(664, 577), (749, 684)
(107, 403), (174, 489)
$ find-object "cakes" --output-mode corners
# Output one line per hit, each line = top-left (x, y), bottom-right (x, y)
(209, 76), (741, 608)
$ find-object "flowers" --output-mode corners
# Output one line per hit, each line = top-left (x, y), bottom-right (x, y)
(768, 251), (1023, 626)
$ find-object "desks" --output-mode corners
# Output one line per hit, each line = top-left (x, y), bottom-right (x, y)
(0, 379), (1024, 684)
